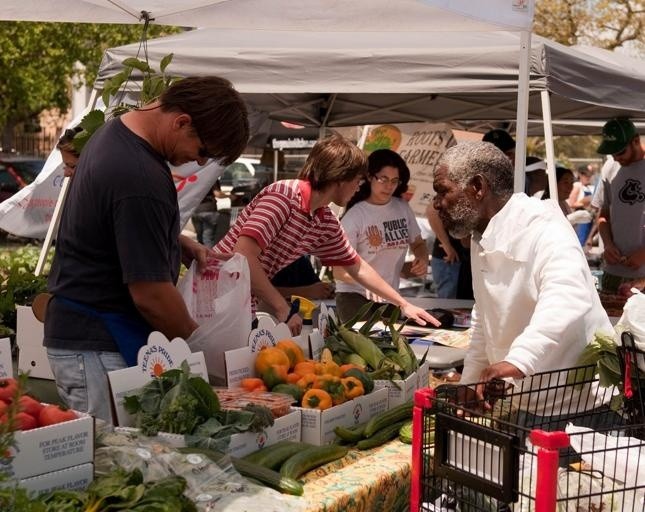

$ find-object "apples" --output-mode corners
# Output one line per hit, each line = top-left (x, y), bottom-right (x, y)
(370, 125), (402, 152)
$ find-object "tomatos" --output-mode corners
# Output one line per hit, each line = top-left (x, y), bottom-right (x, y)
(0, 378), (79, 434)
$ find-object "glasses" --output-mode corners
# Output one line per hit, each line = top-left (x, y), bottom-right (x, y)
(374, 174), (402, 187)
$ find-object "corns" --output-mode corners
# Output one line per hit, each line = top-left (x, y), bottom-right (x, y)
(325, 324), (419, 380)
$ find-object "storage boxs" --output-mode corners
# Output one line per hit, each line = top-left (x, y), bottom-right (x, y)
(373, 358), (430, 404)
(231, 386), (389, 447)
(0, 335), (97, 497)
(113, 410), (301, 459)
(11, 303), (56, 382)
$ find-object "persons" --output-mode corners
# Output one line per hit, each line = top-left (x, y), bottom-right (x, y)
(205, 127), (443, 333)
(432, 138), (623, 441)
(188, 172), (239, 244)
(333, 149), (430, 321)
(43, 76), (249, 435)
(56, 128), (89, 178)
(426, 108), (645, 288)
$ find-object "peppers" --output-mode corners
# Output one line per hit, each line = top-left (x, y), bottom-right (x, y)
(240, 339), (374, 411)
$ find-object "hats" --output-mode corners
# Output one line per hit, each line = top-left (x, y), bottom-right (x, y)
(483, 130), (515, 151)
(597, 117), (639, 154)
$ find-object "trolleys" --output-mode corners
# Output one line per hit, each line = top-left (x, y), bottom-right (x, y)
(409, 330), (645, 511)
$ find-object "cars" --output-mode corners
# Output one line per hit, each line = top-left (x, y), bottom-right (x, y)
(2, 157), (59, 247)
(212, 154), (260, 188)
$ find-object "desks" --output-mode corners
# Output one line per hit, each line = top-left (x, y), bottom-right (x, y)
(1, 321), (472, 511)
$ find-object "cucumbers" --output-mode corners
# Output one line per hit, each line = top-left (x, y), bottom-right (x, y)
(176, 401), (435, 496)
